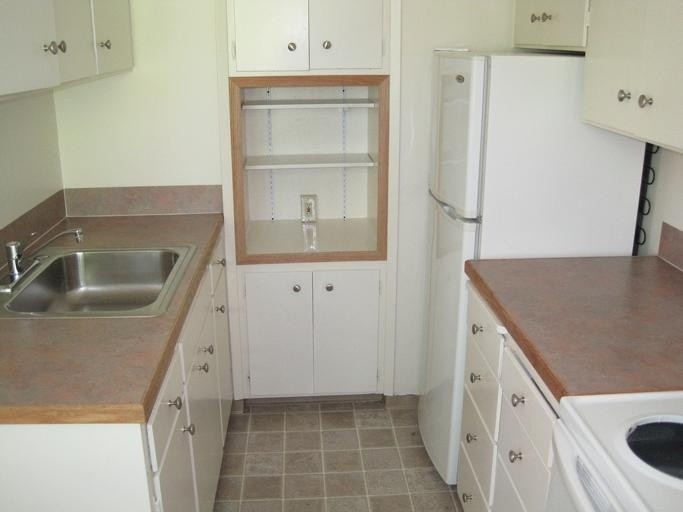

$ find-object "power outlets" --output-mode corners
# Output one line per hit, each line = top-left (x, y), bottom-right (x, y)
(300, 193), (317, 224)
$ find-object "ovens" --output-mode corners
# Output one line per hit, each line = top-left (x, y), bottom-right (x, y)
(538, 421), (626, 512)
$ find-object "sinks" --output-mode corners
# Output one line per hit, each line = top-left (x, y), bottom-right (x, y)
(2, 244), (196, 320)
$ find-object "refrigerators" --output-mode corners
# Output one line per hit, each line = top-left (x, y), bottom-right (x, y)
(415, 48), (661, 490)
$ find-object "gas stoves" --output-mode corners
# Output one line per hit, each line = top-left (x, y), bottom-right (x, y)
(553, 387), (683, 512)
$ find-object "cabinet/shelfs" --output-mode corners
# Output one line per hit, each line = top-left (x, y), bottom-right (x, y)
(439, 280), (502, 511)
(490, 328), (566, 512)
(173, 268), (225, 512)
(1, 349), (201, 511)
(206, 225), (234, 444)
(92, 2), (137, 78)
(509, 1), (588, 54)
(229, 1), (395, 74)
(242, 266), (383, 402)
(238, 94), (376, 172)
(574, 1), (681, 152)
(2, 1), (96, 106)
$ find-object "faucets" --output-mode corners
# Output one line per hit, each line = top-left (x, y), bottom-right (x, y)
(20, 227), (86, 264)
